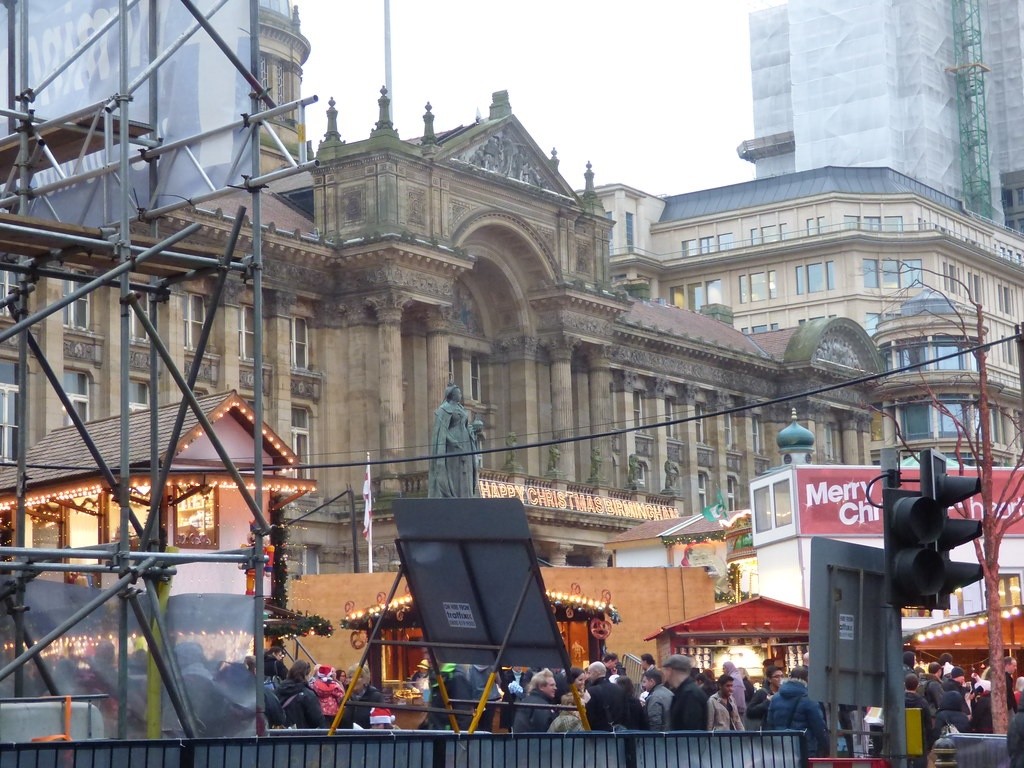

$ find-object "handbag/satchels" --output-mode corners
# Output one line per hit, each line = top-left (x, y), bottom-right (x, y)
(610, 723), (628, 732)
(271, 660), (283, 688)
(940, 712), (959, 736)
(741, 687), (770, 732)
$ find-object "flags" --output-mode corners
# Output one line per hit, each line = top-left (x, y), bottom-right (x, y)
(362, 465), (373, 539)
(701, 491), (728, 522)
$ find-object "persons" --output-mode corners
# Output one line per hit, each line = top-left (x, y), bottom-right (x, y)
(427, 383), (484, 499)
(546, 668), (591, 733)
(903, 651), (992, 751)
(513, 668), (558, 733)
(411, 658), (501, 733)
(661, 655), (710, 733)
(500, 655), (647, 733)
(244, 647), (395, 730)
(340, 663), (384, 729)
(502, 432), (680, 491)
(272, 660), (323, 729)
(639, 651), (756, 732)
(313, 665), (345, 727)
(1005, 656), (1024, 768)
(706, 677), (744, 733)
(263, 647), (289, 681)
(761, 652), (829, 758)
(747, 665), (786, 730)
(20, 633), (283, 741)
(369, 707), (392, 729)
(587, 661), (625, 730)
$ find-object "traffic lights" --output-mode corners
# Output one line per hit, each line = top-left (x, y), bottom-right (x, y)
(881, 487), (945, 610)
(920, 447), (985, 610)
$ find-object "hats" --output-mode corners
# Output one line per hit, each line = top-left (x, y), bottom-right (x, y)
(950, 667), (965, 678)
(174, 642), (204, 663)
(416, 659), (430, 670)
(928, 662), (945, 672)
(317, 665), (332, 682)
(661, 654), (691, 671)
(369, 707), (395, 725)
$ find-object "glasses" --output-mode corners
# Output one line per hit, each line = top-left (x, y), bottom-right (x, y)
(770, 675), (784, 678)
